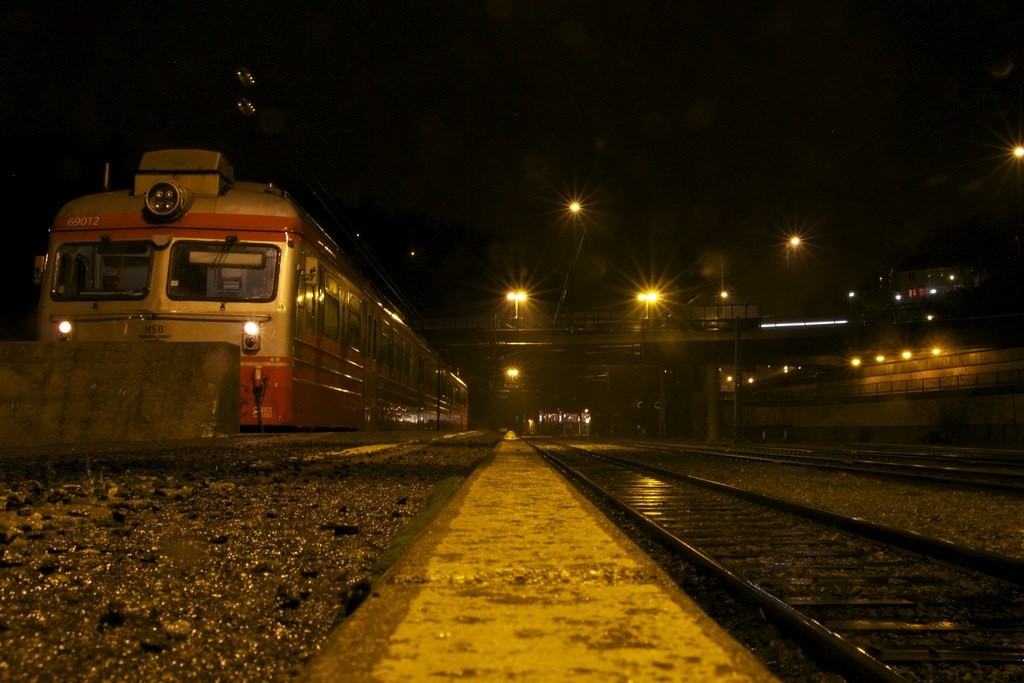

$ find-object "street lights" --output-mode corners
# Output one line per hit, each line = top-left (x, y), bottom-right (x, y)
(564, 193), (583, 327)
(785, 234), (803, 321)
(488, 280), (529, 426)
(720, 280), (744, 441)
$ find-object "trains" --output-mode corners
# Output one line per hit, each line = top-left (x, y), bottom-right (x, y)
(588, 345), (1024, 451)
(33, 148), (468, 436)
